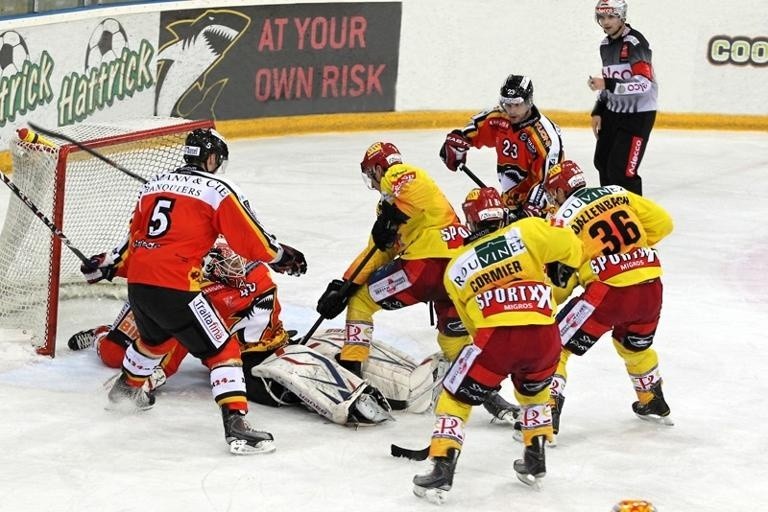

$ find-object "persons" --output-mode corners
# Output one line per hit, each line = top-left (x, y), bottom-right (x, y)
(591, 0), (658, 198)
(411, 185), (583, 504)
(516, 160), (676, 447)
(324, 142), (473, 379)
(67, 128), (450, 453)
(442, 74), (565, 220)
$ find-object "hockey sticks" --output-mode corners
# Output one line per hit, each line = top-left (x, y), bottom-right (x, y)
(392, 443), (430, 460)
(25, 121), (149, 185)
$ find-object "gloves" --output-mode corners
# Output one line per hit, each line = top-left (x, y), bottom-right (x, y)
(546, 261), (575, 288)
(372, 200), (407, 252)
(316, 280), (361, 319)
(268, 243), (306, 277)
(440, 130), (471, 172)
(81, 248), (121, 284)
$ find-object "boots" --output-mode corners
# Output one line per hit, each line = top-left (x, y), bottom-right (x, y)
(514, 435), (546, 477)
(485, 394), (520, 418)
(514, 393), (564, 434)
(633, 377), (670, 416)
(109, 370), (155, 408)
(222, 404), (273, 447)
(414, 449), (460, 491)
(68, 325), (112, 350)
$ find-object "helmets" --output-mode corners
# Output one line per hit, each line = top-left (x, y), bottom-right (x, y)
(202, 233), (247, 288)
(596, 0), (627, 28)
(462, 187), (505, 233)
(500, 75), (533, 116)
(361, 142), (402, 190)
(184, 128), (228, 174)
(543, 161), (586, 205)
